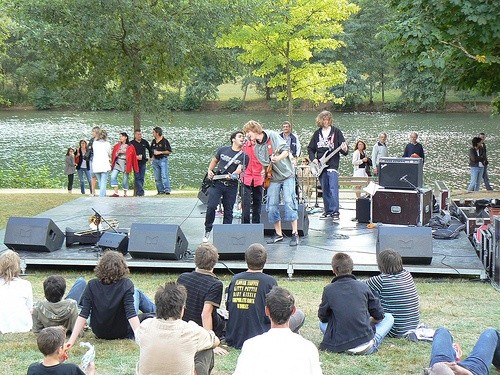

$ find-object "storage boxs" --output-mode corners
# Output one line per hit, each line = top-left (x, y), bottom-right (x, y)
(460, 208), (491, 236)
(451, 199), (476, 214)
(369, 187), (432, 227)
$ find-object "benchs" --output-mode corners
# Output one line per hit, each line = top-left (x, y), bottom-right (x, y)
(308, 177), (379, 199)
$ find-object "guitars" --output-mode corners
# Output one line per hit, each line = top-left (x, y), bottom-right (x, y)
(200, 171), (239, 189)
(308, 142), (349, 177)
(263, 144), (286, 188)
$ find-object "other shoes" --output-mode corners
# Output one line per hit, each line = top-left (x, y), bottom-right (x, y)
(319, 212), (331, 219)
(453, 342), (462, 356)
(266, 232), (283, 243)
(290, 231), (299, 245)
(156, 191), (165, 195)
(332, 212), (340, 223)
(202, 229), (210, 242)
(110, 193), (119, 197)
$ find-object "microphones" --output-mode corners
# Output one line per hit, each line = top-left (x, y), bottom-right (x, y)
(243, 138), (248, 144)
(400, 175), (408, 181)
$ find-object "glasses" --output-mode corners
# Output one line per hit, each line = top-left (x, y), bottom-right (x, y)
(300, 160), (305, 162)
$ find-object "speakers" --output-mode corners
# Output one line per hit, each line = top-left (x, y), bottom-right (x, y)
(127, 223), (189, 260)
(356, 196), (371, 223)
(375, 224), (433, 265)
(3, 217), (65, 252)
(213, 223), (264, 260)
(260, 204), (309, 237)
(378, 158), (423, 189)
(98, 232), (129, 255)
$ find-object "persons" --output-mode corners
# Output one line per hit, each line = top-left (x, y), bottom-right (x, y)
(357, 249), (418, 340)
(110, 132), (139, 196)
(352, 140), (373, 198)
(129, 130), (153, 196)
(176, 244), (229, 341)
(240, 140), (266, 223)
(278, 121), (297, 158)
(231, 286), (323, 375)
(243, 121), (300, 247)
(370, 132), (388, 176)
(0, 249), (33, 334)
(204, 131), (246, 243)
(73, 140), (92, 194)
(83, 126), (106, 196)
(225, 243), (305, 349)
(468, 132), (494, 191)
(134, 282), (220, 375)
(32, 276), (92, 338)
(66, 250), (156, 352)
(64, 147), (77, 191)
(401, 133), (425, 167)
(91, 129), (112, 196)
(308, 110), (348, 220)
(424, 327), (500, 375)
(27, 326), (96, 375)
(151, 127), (172, 194)
(318, 253), (395, 356)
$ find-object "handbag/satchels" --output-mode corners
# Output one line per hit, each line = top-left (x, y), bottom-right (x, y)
(84, 141), (93, 161)
(405, 326), (435, 341)
(75, 148), (80, 164)
